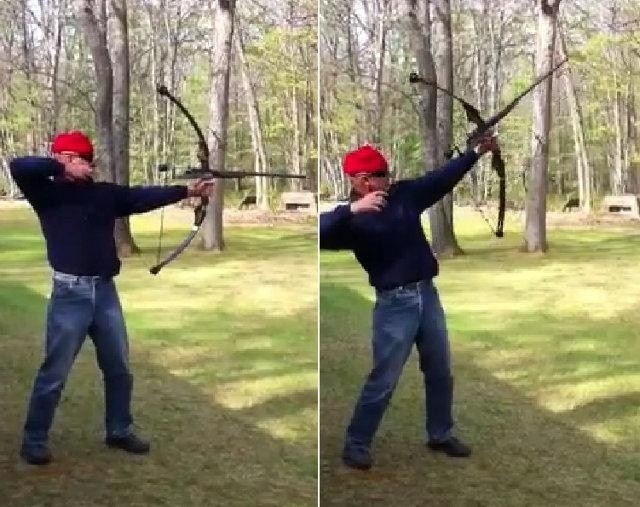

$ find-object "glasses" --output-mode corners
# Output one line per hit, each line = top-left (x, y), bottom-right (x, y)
(369, 171), (386, 179)
(68, 154), (94, 162)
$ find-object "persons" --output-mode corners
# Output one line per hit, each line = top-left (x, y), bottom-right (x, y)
(321, 130), (503, 472)
(8, 131), (217, 465)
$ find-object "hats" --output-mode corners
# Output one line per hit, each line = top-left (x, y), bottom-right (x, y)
(51, 130), (93, 159)
(342, 145), (388, 174)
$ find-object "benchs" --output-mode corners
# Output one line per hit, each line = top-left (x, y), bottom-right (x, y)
(281, 193), (318, 211)
(562, 197), (579, 212)
(604, 195), (640, 213)
(239, 196), (256, 210)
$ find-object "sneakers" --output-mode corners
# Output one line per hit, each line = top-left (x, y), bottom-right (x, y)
(20, 432), (49, 465)
(343, 441), (372, 471)
(105, 432), (150, 453)
(427, 438), (471, 457)
